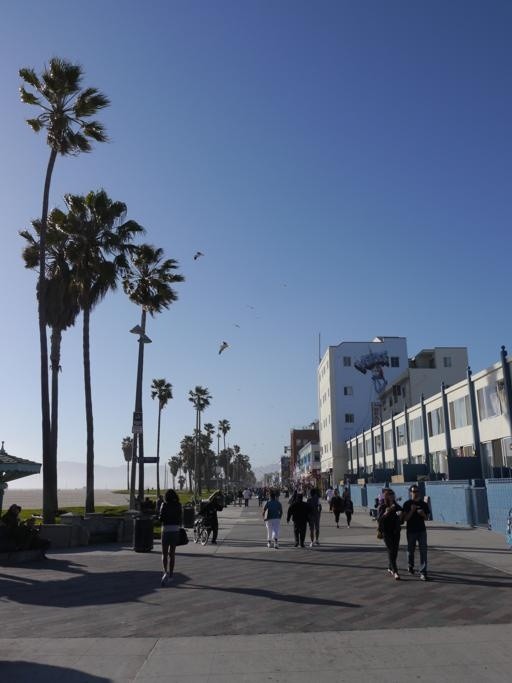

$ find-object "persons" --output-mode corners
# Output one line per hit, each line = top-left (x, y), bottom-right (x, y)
(158, 489), (182, 589)
(197, 495), (222, 544)
(144, 488), (164, 514)
(0, 502), (52, 552)
(373, 487), (406, 580)
(231, 482), (353, 550)
(401, 485), (430, 581)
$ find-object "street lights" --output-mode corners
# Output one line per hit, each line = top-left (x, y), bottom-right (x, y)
(194, 428), (201, 502)
(127, 323), (153, 510)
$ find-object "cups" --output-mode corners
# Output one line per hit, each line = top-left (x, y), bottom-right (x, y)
(416, 508), (424, 514)
(395, 510), (402, 516)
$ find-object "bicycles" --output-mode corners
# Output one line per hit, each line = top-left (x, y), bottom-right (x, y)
(193, 512), (209, 545)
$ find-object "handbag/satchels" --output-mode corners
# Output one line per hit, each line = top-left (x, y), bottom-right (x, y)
(179, 529), (189, 546)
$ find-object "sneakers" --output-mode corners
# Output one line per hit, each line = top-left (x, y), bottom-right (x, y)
(406, 565), (415, 573)
(294, 541), (305, 547)
(265, 541), (280, 549)
(309, 538), (321, 548)
(419, 571), (429, 581)
(160, 571), (168, 587)
(386, 565), (403, 581)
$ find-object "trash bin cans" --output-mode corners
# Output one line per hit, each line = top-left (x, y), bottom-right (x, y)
(132, 515), (154, 553)
(183, 502), (195, 528)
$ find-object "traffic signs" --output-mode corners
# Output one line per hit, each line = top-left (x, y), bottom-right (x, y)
(139, 457), (160, 463)
